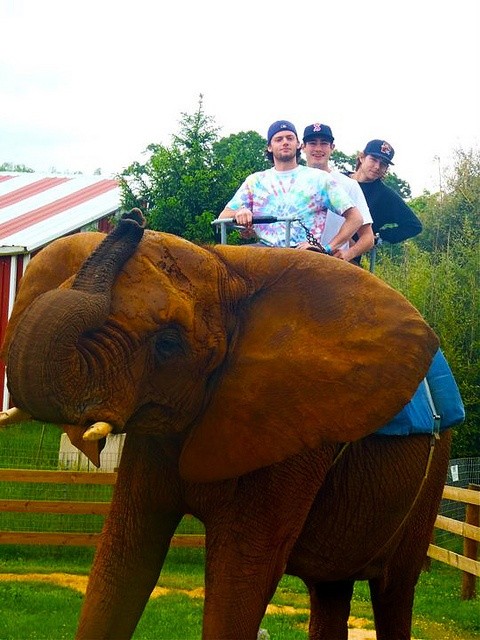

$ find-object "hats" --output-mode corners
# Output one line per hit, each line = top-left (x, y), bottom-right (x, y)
(303, 123), (334, 146)
(363, 140), (395, 166)
(268, 120), (298, 144)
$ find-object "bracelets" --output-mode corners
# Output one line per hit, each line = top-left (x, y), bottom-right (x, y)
(376, 233), (380, 241)
(324, 245), (333, 256)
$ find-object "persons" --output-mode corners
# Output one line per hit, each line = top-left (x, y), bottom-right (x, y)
(339, 139), (422, 265)
(218, 120), (364, 251)
(300, 122), (375, 261)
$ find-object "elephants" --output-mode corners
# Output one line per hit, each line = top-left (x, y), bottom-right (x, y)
(1, 208), (453, 640)
